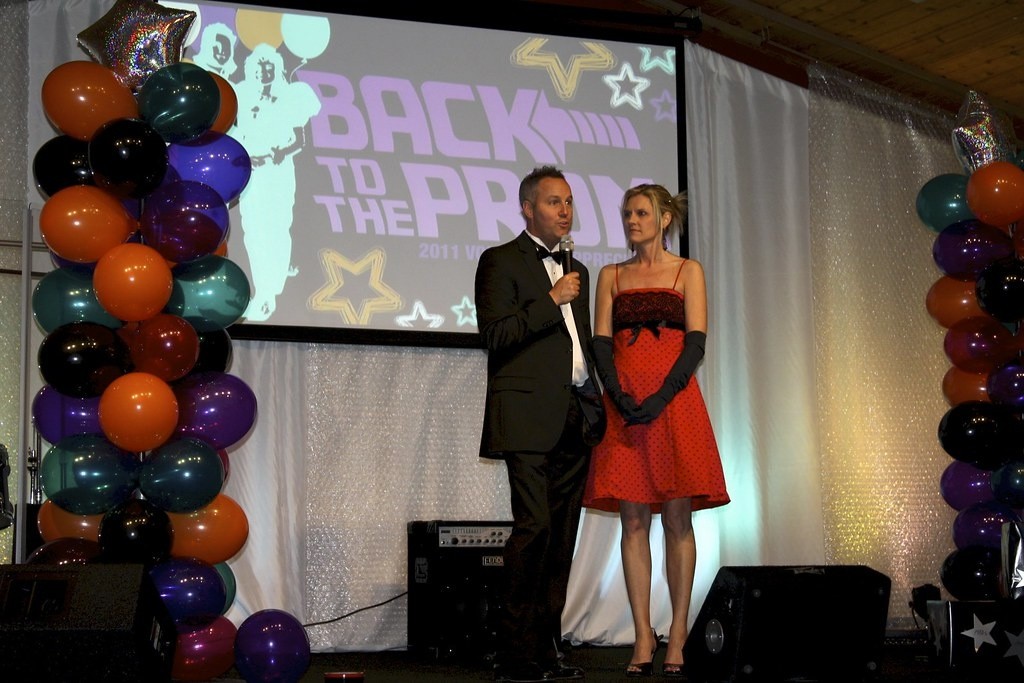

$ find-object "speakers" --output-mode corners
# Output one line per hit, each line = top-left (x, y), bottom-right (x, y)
(408, 520), (514, 673)
(0, 564), (178, 683)
(682, 565), (892, 683)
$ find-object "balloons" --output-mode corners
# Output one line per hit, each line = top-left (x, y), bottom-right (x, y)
(32, 0), (258, 683)
(916, 88), (1024, 597)
(234, 610), (310, 683)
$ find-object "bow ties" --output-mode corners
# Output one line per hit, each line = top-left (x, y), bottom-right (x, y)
(538, 246), (562, 265)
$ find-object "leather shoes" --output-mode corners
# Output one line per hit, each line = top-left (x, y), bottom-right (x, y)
(491, 660), (552, 683)
(540, 662), (585, 680)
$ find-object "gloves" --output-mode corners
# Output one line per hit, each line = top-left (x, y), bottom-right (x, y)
(592, 335), (639, 419)
(624, 331), (707, 428)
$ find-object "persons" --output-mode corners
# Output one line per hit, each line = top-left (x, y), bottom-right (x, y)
(473, 166), (606, 680)
(583, 184), (730, 675)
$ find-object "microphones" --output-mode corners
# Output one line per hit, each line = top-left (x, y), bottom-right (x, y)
(560, 234), (575, 275)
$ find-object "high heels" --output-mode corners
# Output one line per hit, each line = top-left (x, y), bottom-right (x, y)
(627, 629), (661, 677)
(663, 635), (689, 677)
